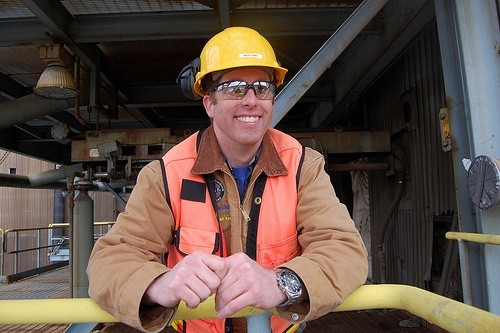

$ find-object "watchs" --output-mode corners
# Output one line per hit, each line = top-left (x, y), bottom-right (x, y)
(272, 267), (302, 307)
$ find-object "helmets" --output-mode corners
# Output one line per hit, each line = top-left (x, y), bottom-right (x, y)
(177, 26), (288, 101)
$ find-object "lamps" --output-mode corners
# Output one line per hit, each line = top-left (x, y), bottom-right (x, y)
(32, 62), (79, 98)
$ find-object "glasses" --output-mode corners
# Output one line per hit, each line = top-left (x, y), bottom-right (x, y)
(208, 80), (276, 100)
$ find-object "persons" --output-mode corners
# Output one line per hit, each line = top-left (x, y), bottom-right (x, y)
(86, 27), (369, 333)
